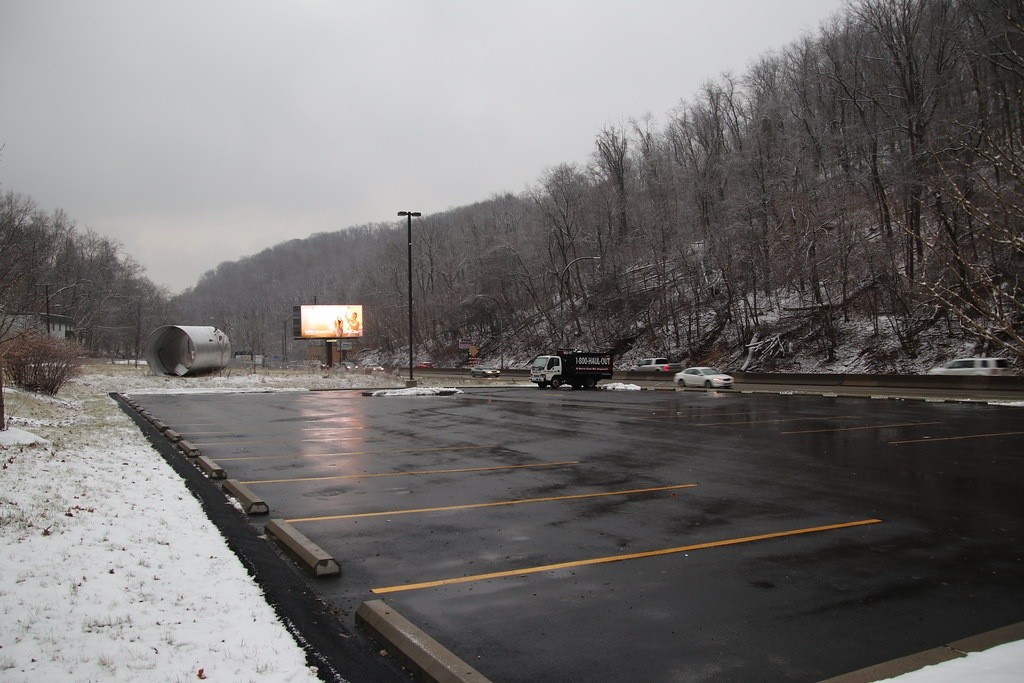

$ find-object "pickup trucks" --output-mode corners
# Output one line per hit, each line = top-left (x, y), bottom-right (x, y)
(629, 357), (680, 373)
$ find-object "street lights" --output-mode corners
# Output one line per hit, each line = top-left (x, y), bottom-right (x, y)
(398, 210), (423, 378)
(557, 256), (600, 348)
(482, 292), (504, 370)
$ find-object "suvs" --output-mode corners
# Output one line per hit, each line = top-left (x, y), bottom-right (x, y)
(927, 357), (1011, 376)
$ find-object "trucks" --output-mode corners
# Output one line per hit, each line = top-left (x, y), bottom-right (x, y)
(528, 350), (617, 391)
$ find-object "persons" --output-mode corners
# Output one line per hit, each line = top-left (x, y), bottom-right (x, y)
(334, 307), (359, 337)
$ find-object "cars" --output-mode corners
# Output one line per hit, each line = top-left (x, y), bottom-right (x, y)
(289, 361), (386, 374)
(674, 365), (736, 389)
(471, 365), (503, 377)
